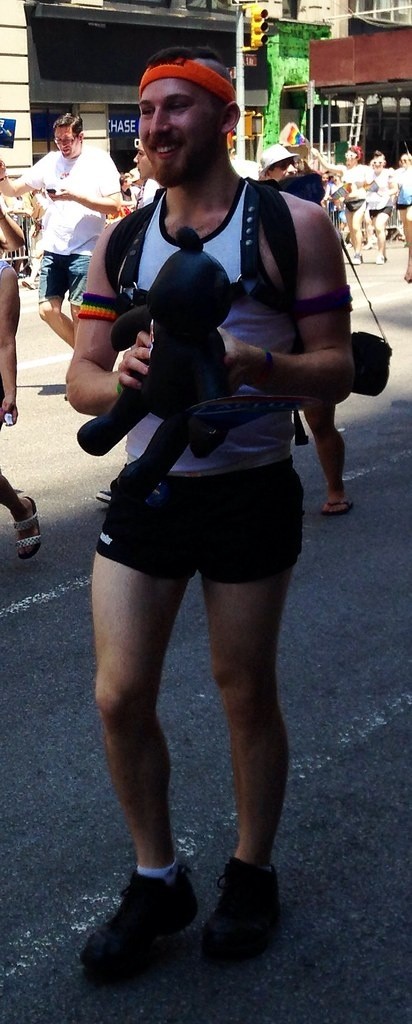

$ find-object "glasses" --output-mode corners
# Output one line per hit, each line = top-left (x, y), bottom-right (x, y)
(54, 134), (78, 144)
(134, 139), (142, 149)
(345, 154), (354, 158)
(271, 160), (299, 171)
(373, 161), (383, 164)
(401, 160), (410, 163)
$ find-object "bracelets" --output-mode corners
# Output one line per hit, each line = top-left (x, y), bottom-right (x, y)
(262, 349), (272, 381)
(116, 383), (123, 396)
(1, 176), (7, 182)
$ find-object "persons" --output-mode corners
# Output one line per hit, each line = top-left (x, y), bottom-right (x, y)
(0, 113), (411, 560)
(64, 48), (359, 966)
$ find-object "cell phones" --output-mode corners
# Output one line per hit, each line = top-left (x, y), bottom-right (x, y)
(46, 188), (56, 195)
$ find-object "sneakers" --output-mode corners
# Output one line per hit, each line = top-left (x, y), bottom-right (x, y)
(80, 861), (198, 970)
(202, 854), (279, 964)
(376, 255), (386, 265)
(351, 255), (362, 265)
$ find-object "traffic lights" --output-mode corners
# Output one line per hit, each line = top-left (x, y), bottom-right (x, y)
(244, 113), (265, 136)
(250, 5), (270, 47)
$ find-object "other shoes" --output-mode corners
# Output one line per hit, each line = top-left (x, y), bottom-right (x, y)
(96, 488), (112, 504)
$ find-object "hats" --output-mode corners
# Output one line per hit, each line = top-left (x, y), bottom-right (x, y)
(259, 143), (300, 176)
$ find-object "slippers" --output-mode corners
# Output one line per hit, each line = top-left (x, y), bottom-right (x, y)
(14, 495), (41, 560)
(322, 499), (353, 516)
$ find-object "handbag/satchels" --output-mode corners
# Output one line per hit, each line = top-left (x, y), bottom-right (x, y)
(349, 331), (392, 396)
(342, 199), (366, 212)
